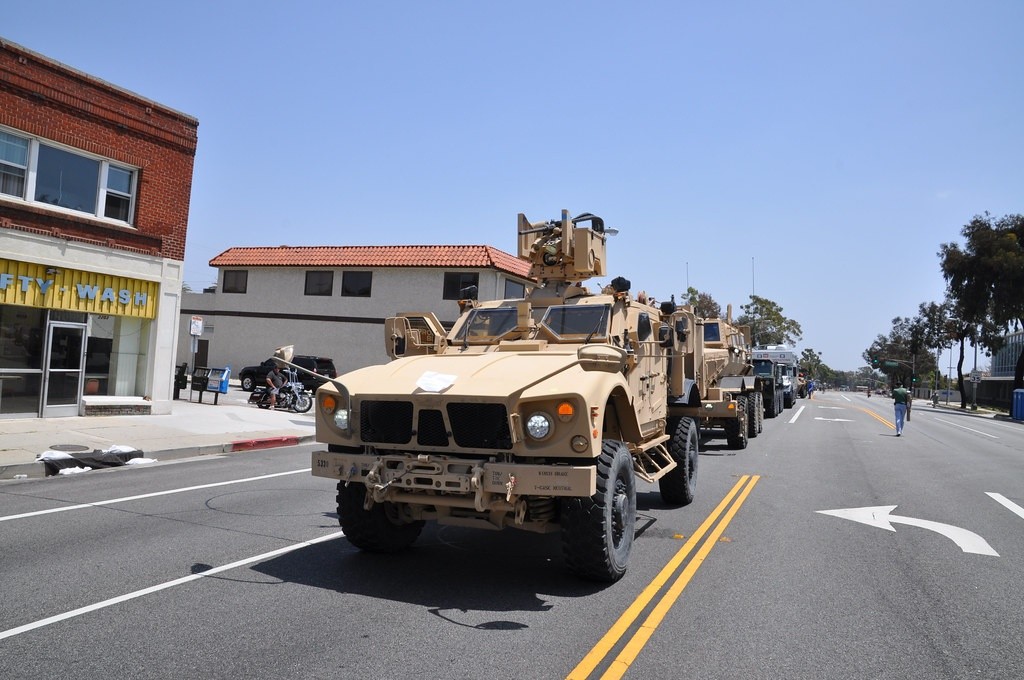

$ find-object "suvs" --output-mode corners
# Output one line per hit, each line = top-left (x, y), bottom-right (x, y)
(239, 355), (338, 395)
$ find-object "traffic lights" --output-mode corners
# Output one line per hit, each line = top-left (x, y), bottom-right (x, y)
(874, 356), (877, 362)
(912, 377), (916, 381)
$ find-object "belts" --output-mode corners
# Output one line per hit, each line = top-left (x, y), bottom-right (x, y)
(899, 403), (906, 405)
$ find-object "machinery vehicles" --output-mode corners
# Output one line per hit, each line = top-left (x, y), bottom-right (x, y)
(312, 209), (812, 583)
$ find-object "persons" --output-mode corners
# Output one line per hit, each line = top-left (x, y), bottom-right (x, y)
(929, 391), (938, 408)
(891, 381), (912, 437)
(265, 365), (288, 410)
(807, 380), (814, 399)
(868, 388), (871, 398)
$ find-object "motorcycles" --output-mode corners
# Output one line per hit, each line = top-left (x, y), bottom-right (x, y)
(248, 383), (313, 413)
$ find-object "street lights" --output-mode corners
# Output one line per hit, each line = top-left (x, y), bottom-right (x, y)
(947, 317), (976, 411)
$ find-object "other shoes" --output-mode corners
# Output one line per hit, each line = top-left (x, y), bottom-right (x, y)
(270, 407), (274, 411)
(288, 408), (296, 411)
(897, 434), (901, 436)
